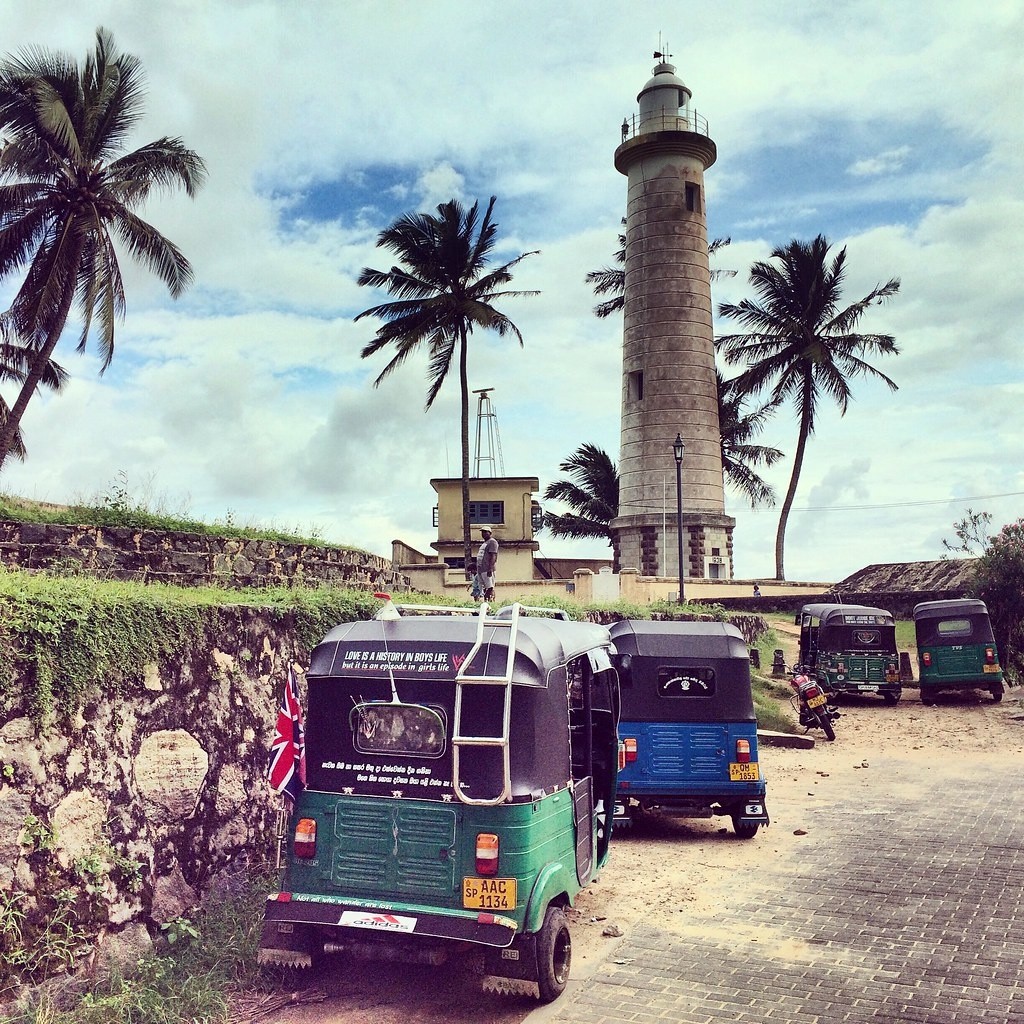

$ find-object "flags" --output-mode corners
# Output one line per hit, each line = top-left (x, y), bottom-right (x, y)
(263, 662), (303, 800)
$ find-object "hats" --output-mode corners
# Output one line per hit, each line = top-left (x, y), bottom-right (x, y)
(479, 526), (492, 535)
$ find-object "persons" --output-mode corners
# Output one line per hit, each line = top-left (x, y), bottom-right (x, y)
(476, 526), (499, 602)
(466, 562), (481, 602)
(753, 585), (760, 596)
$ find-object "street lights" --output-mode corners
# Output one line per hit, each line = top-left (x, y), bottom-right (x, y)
(670, 432), (687, 605)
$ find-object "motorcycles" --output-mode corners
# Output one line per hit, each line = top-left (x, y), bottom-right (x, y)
(770, 653), (840, 741)
(254, 592), (627, 1006)
(592, 619), (771, 842)
(913, 599), (1005, 706)
(795, 603), (904, 706)
